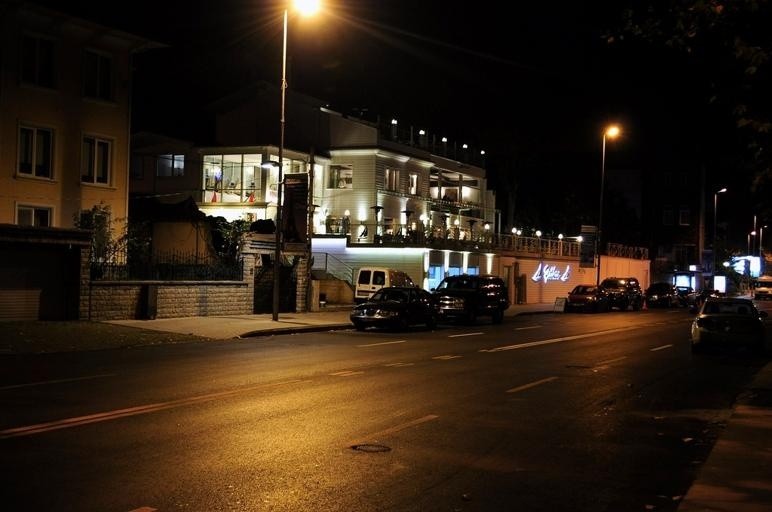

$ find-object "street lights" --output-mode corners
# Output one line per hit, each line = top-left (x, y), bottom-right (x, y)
(596, 122), (624, 288)
(712, 187), (728, 278)
(759, 225), (768, 276)
(272, 2), (329, 323)
(742, 230), (756, 283)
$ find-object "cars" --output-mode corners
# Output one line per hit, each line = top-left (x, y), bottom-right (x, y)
(644, 282), (722, 308)
(691, 297), (769, 349)
(566, 285), (611, 310)
(350, 287), (441, 333)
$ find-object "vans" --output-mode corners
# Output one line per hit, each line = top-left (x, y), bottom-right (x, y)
(355, 265), (413, 300)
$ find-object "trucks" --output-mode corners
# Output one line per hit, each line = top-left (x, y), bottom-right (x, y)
(752, 277), (772, 300)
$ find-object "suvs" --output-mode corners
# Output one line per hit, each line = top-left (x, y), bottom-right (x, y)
(430, 274), (510, 322)
(601, 275), (645, 312)
